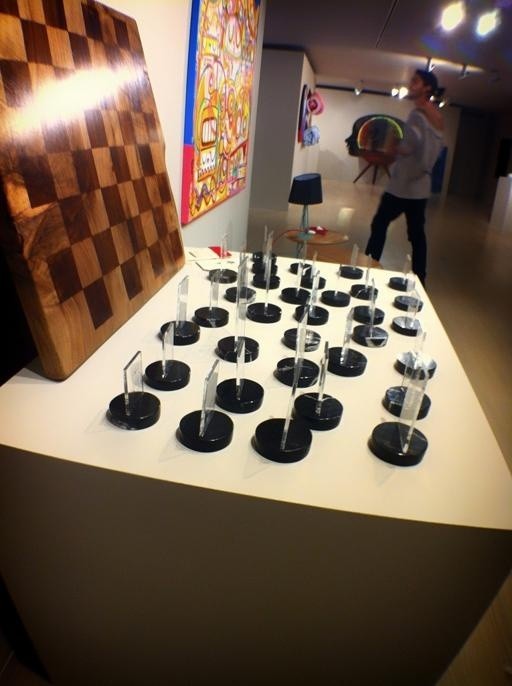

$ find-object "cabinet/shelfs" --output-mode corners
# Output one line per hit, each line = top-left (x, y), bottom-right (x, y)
(1, 247), (511, 684)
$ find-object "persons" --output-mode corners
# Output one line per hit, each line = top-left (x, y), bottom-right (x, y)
(366, 68), (446, 287)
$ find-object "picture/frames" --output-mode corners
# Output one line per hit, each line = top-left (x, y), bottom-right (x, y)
(180, 1), (262, 229)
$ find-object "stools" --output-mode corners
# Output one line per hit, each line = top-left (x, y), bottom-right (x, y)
(284, 227), (350, 260)
(304, 244), (382, 268)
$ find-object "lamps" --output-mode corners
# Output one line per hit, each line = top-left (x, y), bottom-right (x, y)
(289, 173), (323, 258)
